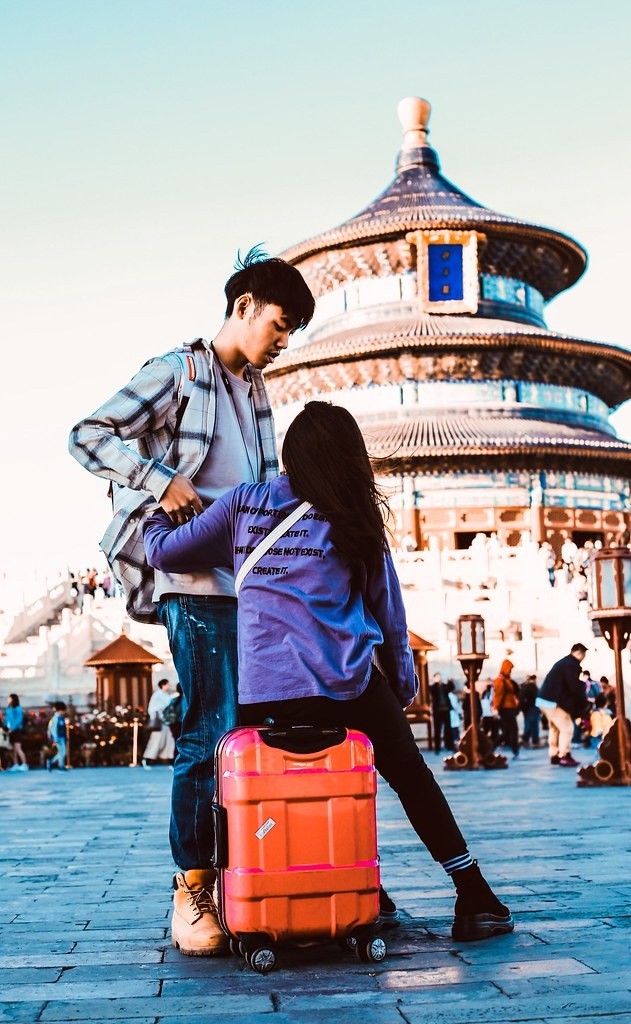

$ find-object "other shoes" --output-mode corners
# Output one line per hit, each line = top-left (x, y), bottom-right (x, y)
(142, 760), (152, 771)
(58, 766), (67, 771)
(521, 739), (530, 749)
(551, 755), (561, 765)
(8, 763), (20, 772)
(560, 753), (581, 765)
(18, 763), (29, 771)
(532, 743), (542, 748)
(46, 759), (53, 771)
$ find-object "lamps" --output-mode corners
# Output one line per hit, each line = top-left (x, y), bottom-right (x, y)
(585, 546), (631, 617)
(457, 612), (487, 661)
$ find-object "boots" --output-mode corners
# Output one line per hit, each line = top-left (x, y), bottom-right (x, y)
(450, 858), (515, 942)
(378, 877), (397, 917)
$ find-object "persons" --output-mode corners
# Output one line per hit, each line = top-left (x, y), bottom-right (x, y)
(0, 569), (618, 772)
(68, 242), (315, 957)
(141, 401), (515, 941)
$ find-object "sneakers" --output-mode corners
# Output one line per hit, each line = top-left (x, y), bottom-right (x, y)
(171, 867), (227, 955)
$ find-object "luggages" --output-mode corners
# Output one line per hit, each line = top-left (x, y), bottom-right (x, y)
(212, 717), (387, 972)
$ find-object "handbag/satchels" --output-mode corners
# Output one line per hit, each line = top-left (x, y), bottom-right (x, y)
(148, 712), (161, 731)
